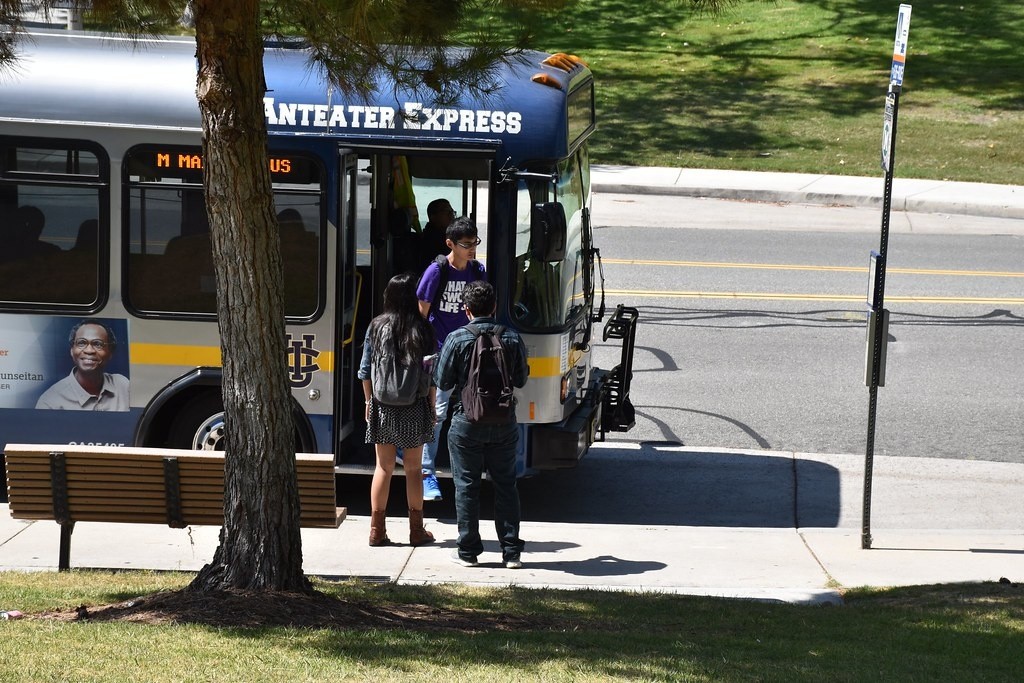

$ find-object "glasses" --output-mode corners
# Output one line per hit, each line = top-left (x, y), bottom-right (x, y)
(73, 338), (112, 351)
(455, 236), (481, 249)
(439, 210), (456, 218)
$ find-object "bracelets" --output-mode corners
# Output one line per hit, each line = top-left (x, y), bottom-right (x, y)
(365, 401), (370, 405)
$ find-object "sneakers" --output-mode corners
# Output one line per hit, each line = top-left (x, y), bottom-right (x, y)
(422, 476), (443, 501)
(503, 559), (521, 569)
(450, 549), (478, 567)
(395, 448), (405, 465)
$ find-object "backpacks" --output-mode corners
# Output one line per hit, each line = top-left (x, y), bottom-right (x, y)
(461, 322), (515, 425)
(370, 320), (432, 406)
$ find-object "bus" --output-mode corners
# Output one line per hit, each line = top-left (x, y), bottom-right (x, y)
(1, 23), (638, 479)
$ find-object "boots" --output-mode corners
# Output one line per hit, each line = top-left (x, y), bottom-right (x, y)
(409, 508), (433, 546)
(368, 511), (390, 546)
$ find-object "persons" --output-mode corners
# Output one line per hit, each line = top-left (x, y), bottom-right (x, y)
(415, 217), (485, 500)
(420, 198), (457, 273)
(437, 280), (528, 569)
(76, 219), (99, 253)
(481, 462), (487, 501)
(16, 206), (59, 260)
(34, 319), (129, 411)
(357, 274), (439, 549)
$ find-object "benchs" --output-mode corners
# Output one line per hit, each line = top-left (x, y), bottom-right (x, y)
(5, 444), (347, 572)
